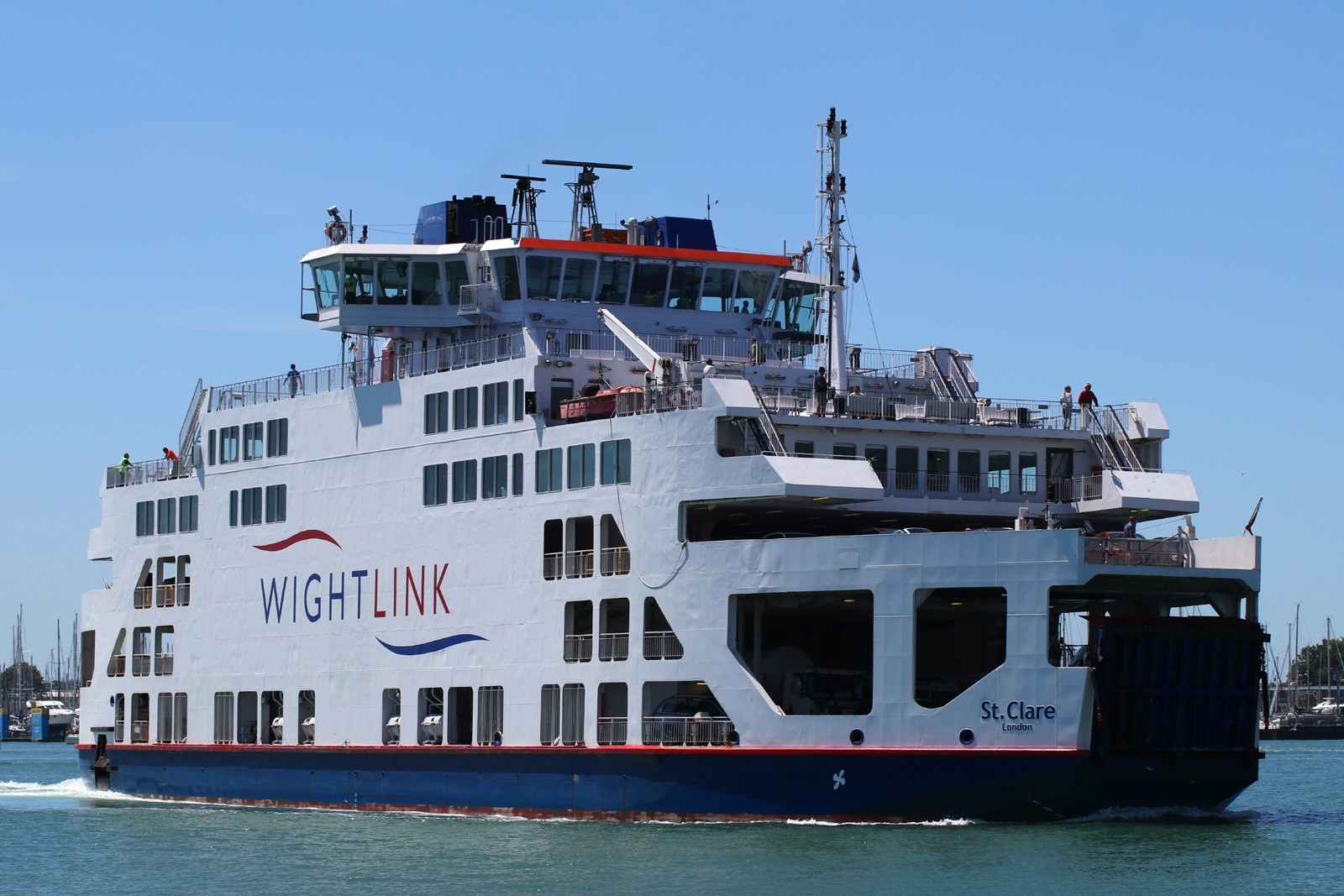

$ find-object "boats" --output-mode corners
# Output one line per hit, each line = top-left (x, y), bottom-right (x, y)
(25, 699), (77, 726)
(559, 383), (648, 423)
(50, 95), (1273, 825)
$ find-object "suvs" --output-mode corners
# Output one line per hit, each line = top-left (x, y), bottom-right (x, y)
(650, 693), (734, 745)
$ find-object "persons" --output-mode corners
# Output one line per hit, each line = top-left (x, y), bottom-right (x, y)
(119, 453), (134, 486)
(162, 447), (178, 475)
(749, 336), (763, 364)
(815, 367), (829, 417)
(849, 385), (864, 396)
(283, 363), (302, 398)
(345, 271), (358, 303)
(1123, 516), (1136, 563)
(1078, 382), (1099, 427)
(1060, 385), (1073, 430)
(742, 301), (749, 313)
(703, 358), (718, 378)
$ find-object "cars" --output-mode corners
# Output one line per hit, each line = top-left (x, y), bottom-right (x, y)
(1149, 536), (1199, 567)
(856, 527), (934, 533)
(760, 530), (817, 539)
(1086, 531), (1148, 564)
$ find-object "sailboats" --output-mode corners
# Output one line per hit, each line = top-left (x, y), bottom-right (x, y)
(1258, 602), (1344, 730)
(1, 603), (79, 745)
